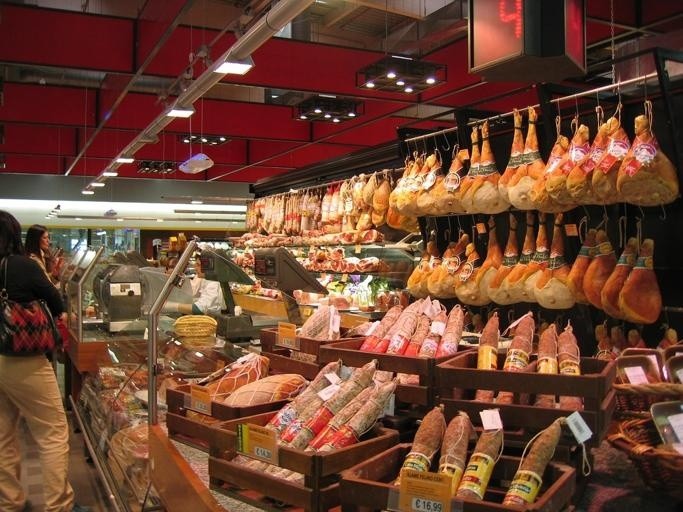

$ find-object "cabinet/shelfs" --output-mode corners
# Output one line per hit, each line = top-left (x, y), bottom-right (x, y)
(396, 45), (682, 357)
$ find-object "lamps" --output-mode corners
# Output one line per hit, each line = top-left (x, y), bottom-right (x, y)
(81, 9), (257, 195)
(292, 95), (364, 125)
(355, 0), (447, 97)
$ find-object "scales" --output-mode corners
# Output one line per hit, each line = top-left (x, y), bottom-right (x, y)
(114, 250), (128, 264)
(128, 250), (154, 268)
(252, 246), (329, 326)
(198, 247), (291, 338)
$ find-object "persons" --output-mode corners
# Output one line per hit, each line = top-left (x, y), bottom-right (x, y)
(159, 254), (228, 318)
(1, 211), (91, 512)
(24, 224), (62, 295)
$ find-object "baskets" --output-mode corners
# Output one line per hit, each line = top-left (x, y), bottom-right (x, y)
(611, 376), (683, 419)
(606, 414), (683, 502)
(172, 314), (218, 338)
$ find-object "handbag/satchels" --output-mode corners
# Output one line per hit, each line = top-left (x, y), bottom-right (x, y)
(0, 297), (63, 357)
